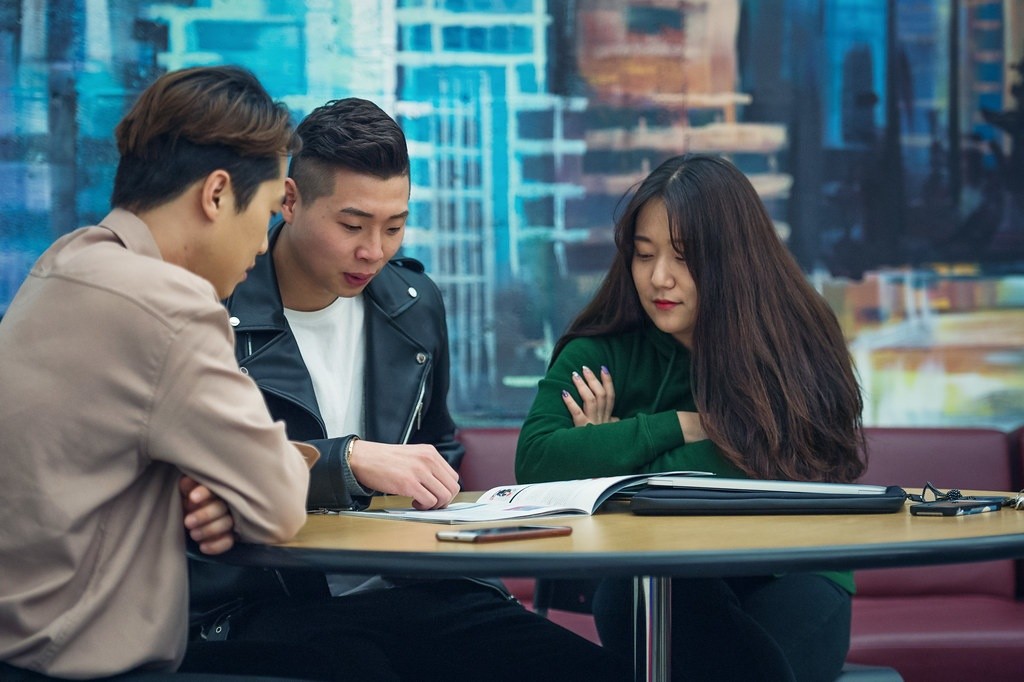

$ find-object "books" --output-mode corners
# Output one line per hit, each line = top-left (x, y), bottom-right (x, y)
(339, 472), (716, 523)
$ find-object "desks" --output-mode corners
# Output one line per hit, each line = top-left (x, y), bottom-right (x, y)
(172, 483), (1023, 681)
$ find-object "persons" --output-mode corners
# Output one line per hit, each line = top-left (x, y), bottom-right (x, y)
(0, 65), (321, 682)
(514, 151), (869, 682)
(180, 98), (644, 682)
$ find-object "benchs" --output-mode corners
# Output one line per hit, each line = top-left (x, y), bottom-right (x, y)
(432, 425), (1023, 679)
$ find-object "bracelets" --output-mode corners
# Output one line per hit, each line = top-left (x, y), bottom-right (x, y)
(348, 437), (359, 464)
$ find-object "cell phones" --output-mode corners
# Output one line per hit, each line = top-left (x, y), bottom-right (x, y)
(910, 498), (1002, 517)
(435, 525), (573, 545)
